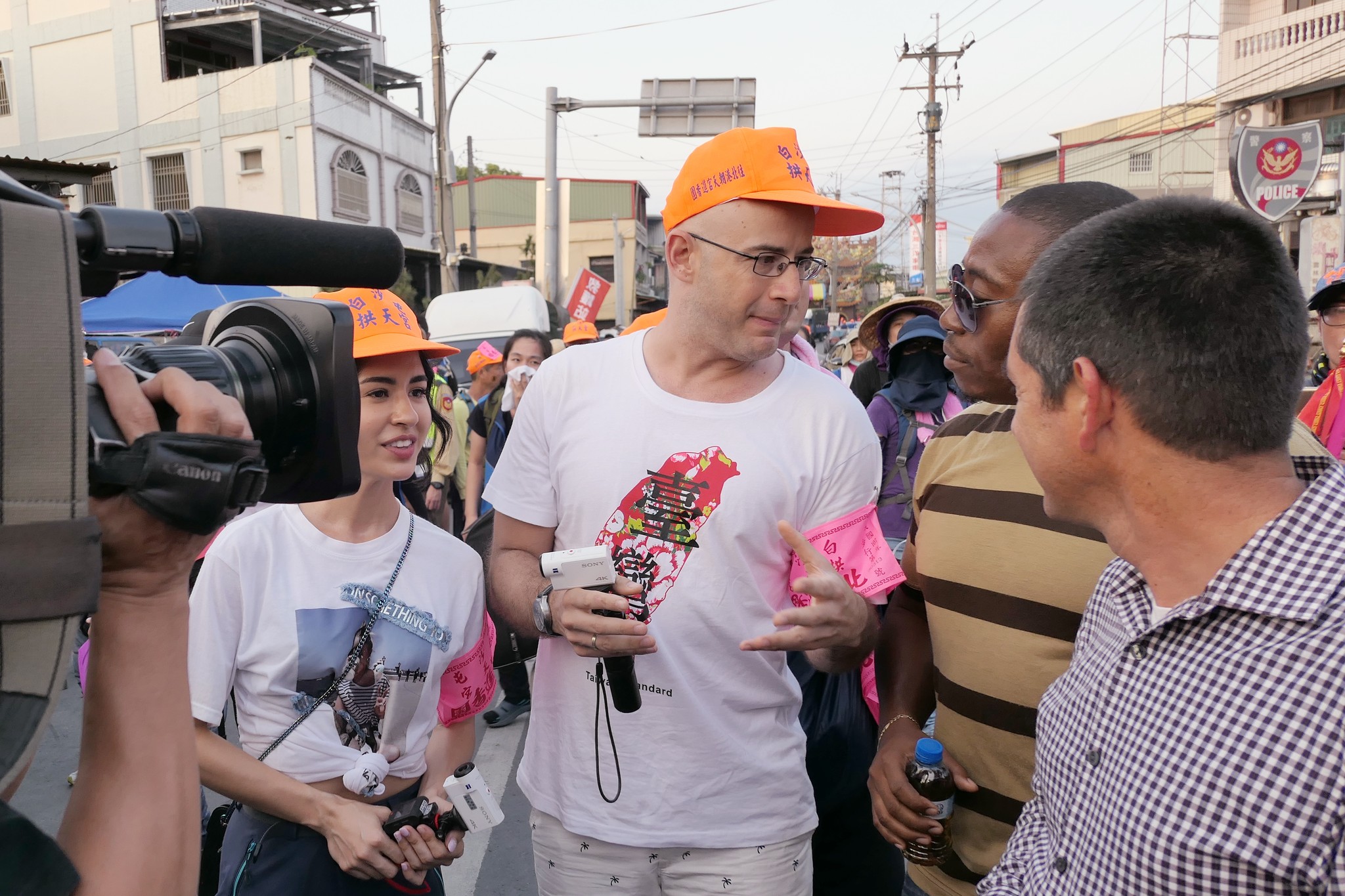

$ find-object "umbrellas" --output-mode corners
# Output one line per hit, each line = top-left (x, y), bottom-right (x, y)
(80, 271), (295, 337)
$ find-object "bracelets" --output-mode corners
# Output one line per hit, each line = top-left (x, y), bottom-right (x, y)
(875, 714), (923, 751)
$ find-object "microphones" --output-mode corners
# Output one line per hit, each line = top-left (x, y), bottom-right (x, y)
(69, 206), (404, 292)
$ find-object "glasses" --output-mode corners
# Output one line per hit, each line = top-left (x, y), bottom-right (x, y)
(1319, 306), (1345, 326)
(687, 233), (828, 280)
(946, 263), (1019, 333)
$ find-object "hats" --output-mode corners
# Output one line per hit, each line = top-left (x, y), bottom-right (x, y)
(857, 292), (946, 352)
(562, 320), (600, 343)
(311, 287), (461, 359)
(887, 315), (948, 352)
(871, 305), (941, 372)
(1306, 262), (1345, 310)
(846, 331), (859, 344)
(466, 348), (503, 374)
(619, 307), (669, 335)
(660, 126), (885, 238)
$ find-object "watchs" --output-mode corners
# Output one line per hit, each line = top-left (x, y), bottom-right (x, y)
(431, 482), (444, 489)
(532, 584), (564, 638)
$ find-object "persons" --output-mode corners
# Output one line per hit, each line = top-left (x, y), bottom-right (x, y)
(461, 330), (555, 728)
(864, 315), (965, 742)
(478, 125), (889, 896)
(184, 287), (487, 896)
(394, 313), (504, 545)
(619, 279), (948, 408)
(970, 193), (1345, 896)
(549, 320), (625, 358)
(1300, 262), (1345, 388)
(0, 346), (255, 896)
(863, 182), (1147, 896)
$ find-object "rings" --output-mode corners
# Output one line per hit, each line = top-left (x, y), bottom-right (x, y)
(591, 634), (599, 651)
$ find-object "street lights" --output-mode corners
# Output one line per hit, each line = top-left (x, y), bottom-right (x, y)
(432, 47), (499, 293)
(849, 188), (937, 301)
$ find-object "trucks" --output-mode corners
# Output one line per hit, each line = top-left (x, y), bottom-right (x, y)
(802, 308), (829, 342)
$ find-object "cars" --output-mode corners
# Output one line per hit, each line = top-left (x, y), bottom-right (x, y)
(823, 330), (847, 355)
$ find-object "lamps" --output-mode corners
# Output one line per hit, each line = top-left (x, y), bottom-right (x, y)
(238, 5), (246, 13)
(215, 6), (225, 16)
(169, 12), (175, 21)
(190, 8), (198, 19)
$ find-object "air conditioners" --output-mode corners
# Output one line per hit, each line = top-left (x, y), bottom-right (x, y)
(1234, 99), (1273, 129)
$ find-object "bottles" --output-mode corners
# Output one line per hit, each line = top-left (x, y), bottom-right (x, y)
(901, 737), (956, 866)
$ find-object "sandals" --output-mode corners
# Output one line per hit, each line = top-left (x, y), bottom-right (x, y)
(482, 696), (531, 727)
